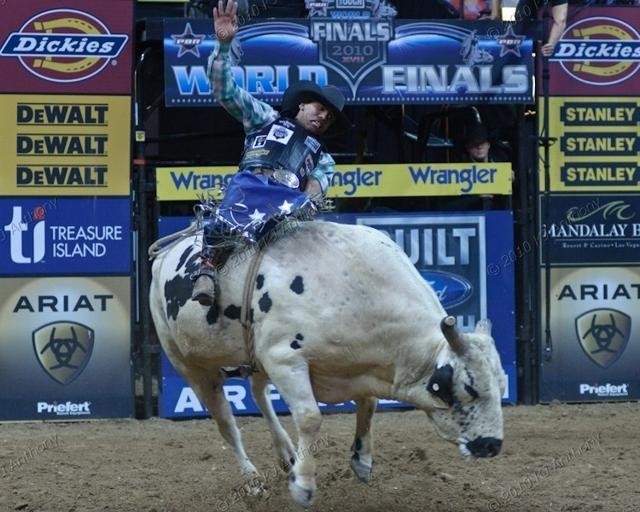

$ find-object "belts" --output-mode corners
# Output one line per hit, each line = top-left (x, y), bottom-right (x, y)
(250, 167), (301, 192)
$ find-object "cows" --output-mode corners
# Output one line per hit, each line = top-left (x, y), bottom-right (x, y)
(148, 219), (507, 509)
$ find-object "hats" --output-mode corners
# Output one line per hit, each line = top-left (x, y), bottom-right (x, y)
(282, 80), (353, 136)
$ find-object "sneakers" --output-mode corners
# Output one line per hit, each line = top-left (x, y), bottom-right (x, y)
(192, 261), (215, 306)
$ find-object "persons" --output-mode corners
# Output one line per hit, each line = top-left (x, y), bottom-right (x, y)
(191, 0), (352, 307)
(391, 0), (568, 210)
